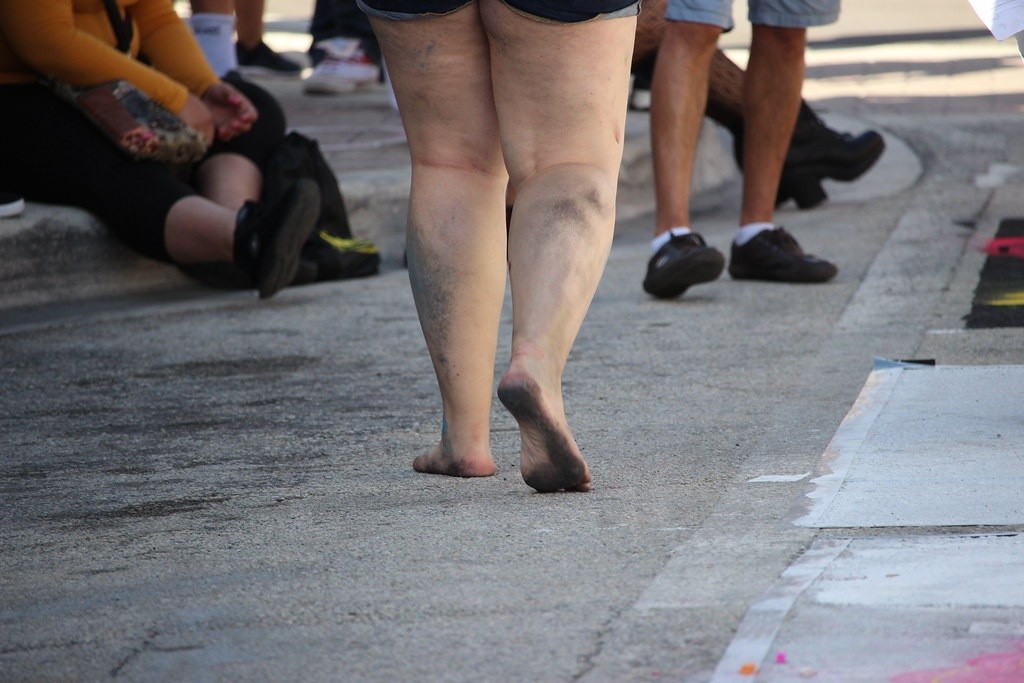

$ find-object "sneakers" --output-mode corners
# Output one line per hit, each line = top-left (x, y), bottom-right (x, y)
(643, 233), (725, 300)
(729, 228), (839, 283)
(234, 173), (320, 296)
(238, 42), (301, 73)
(305, 56), (383, 93)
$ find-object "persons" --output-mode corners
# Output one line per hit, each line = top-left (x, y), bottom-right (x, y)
(0, 0), (323, 301)
(629, 0), (888, 212)
(642, 0), (840, 298)
(189, 0), (301, 79)
(359, 0), (642, 494)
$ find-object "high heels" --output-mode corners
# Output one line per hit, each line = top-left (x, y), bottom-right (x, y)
(734, 100), (884, 208)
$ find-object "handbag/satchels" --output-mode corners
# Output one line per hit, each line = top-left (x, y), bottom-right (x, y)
(294, 227), (381, 282)
(72, 78), (206, 164)
